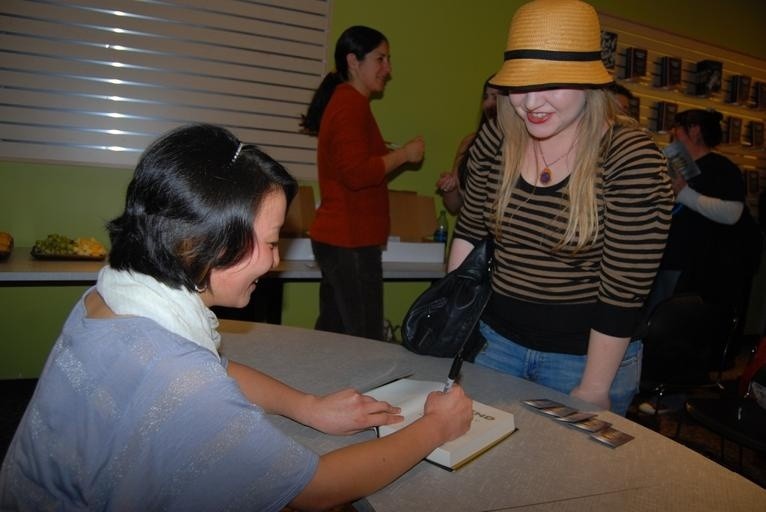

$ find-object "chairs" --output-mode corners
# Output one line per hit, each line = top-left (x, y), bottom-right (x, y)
(631, 203), (753, 428)
(681, 326), (766, 471)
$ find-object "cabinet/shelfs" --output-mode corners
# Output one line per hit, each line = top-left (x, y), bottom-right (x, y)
(597, 15), (765, 176)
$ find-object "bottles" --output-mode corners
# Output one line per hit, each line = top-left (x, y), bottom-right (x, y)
(434, 210), (449, 242)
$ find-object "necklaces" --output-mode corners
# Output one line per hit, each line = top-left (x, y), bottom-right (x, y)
(536, 139), (571, 187)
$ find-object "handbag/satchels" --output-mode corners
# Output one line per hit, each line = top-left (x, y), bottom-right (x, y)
(401, 234), (493, 357)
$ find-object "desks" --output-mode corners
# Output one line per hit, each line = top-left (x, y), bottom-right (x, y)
(211, 316), (766, 511)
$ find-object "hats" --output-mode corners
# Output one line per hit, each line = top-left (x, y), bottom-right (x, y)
(485, 1), (615, 91)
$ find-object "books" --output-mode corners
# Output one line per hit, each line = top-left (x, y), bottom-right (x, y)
(660, 142), (700, 183)
(349, 379), (518, 469)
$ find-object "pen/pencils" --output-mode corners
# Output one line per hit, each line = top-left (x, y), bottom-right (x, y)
(443, 353), (464, 394)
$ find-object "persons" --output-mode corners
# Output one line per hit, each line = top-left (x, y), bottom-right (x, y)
(613, 85), (632, 111)
(437, 74), (502, 215)
(300, 25), (426, 342)
(447, 0), (675, 414)
(2, 126), (473, 511)
(635, 110), (747, 416)
